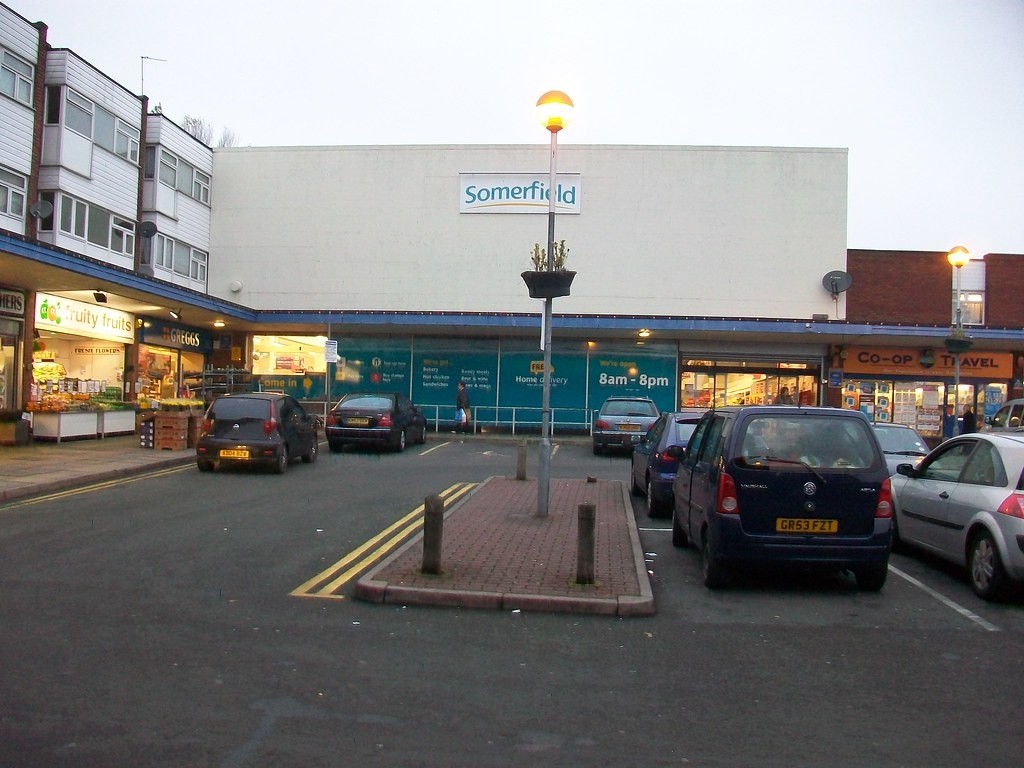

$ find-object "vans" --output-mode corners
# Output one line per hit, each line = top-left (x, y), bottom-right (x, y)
(972, 397), (1023, 433)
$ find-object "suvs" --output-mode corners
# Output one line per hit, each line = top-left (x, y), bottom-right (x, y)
(662, 403), (896, 594)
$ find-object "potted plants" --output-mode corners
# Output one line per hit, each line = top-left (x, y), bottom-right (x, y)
(522, 239), (577, 298)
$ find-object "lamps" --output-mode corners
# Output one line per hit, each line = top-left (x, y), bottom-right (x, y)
(93, 292), (108, 303)
(170, 308), (182, 321)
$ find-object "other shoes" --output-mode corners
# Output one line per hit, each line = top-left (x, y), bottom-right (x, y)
(451, 430), (456, 433)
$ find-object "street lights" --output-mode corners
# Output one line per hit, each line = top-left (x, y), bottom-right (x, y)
(536, 90), (577, 517)
(946, 246), (973, 438)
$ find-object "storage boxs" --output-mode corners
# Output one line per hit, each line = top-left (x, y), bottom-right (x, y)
(139, 410), (202, 451)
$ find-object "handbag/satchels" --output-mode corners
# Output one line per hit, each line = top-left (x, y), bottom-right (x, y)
(455, 409), (462, 421)
(458, 410), (466, 423)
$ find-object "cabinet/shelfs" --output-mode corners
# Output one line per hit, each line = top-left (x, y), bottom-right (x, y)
(181, 365), (253, 399)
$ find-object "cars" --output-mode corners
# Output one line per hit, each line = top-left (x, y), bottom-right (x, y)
(630, 410), (708, 519)
(195, 392), (319, 474)
(325, 392), (428, 452)
(592, 395), (662, 457)
(889, 431), (1024, 602)
(844, 421), (941, 478)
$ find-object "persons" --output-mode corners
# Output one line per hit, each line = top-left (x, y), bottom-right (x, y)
(943, 405), (963, 455)
(775, 387), (793, 404)
(960, 404), (977, 454)
(451, 381), (470, 434)
